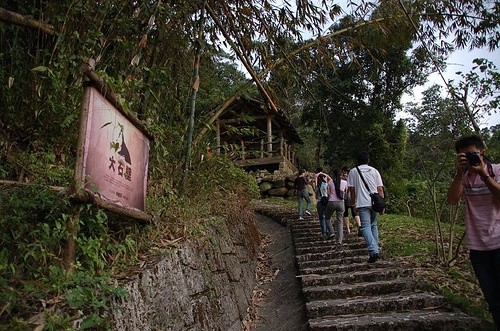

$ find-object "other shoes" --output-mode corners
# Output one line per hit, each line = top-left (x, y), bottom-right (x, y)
(358, 226), (362, 237)
(322, 230), (327, 234)
(328, 233), (335, 239)
(345, 231), (351, 236)
(367, 253), (379, 263)
(337, 242), (342, 245)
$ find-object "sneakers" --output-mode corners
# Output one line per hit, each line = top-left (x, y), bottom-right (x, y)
(305, 210), (311, 215)
(299, 217), (304, 220)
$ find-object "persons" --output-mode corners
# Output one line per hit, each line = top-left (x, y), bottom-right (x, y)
(447, 135), (500, 331)
(347, 151), (386, 264)
(294, 169), (312, 220)
(311, 167), (361, 245)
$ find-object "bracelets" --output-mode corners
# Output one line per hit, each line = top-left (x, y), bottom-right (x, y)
(352, 204), (356, 206)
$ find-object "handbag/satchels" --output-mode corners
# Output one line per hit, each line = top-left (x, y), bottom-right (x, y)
(369, 193), (385, 212)
(339, 191), (348, 199)
(322, 196), (328, 205)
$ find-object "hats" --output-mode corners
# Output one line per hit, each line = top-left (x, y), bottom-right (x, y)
(356, 151), (369, 160)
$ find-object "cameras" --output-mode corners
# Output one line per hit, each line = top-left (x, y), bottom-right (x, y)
(459, 151), (481, 167)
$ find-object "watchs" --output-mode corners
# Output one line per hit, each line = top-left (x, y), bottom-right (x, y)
(480, 174), (489, 181)
